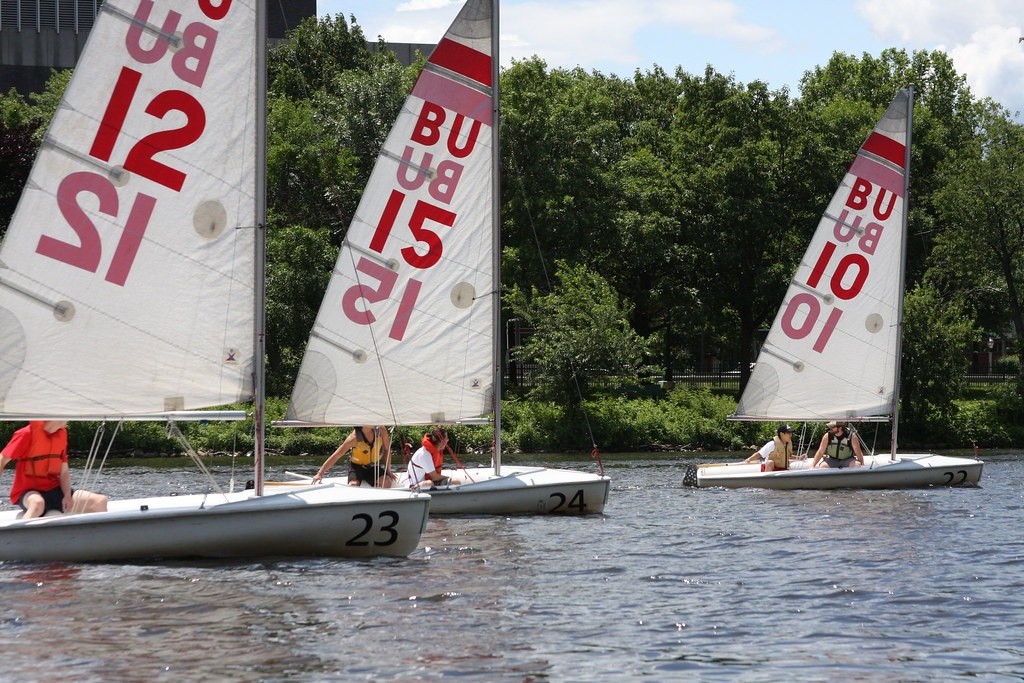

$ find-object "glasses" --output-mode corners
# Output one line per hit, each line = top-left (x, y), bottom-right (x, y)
(445, 438), (450, 443)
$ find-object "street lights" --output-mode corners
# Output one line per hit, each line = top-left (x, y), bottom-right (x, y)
(986, 337), (994, 375)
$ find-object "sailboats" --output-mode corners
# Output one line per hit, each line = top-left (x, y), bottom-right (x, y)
(0, 0), (431, 560)
(685, 83), (984, 488)
(246, 0), (611, 514)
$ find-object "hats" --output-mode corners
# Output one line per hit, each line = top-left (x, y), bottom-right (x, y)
(776, 424), (795, 432)
(825, 421), (845, 429)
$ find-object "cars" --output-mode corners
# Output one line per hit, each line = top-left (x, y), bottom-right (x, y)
(717, 363), (756, 376)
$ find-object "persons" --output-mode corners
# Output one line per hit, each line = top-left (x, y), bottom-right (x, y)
(0, 420), (108, 521)
(408, 428), (462, 493)
(811, 420), (865, 469)
(744, 424), (807, 472)
(311, 425), (399, 489)
